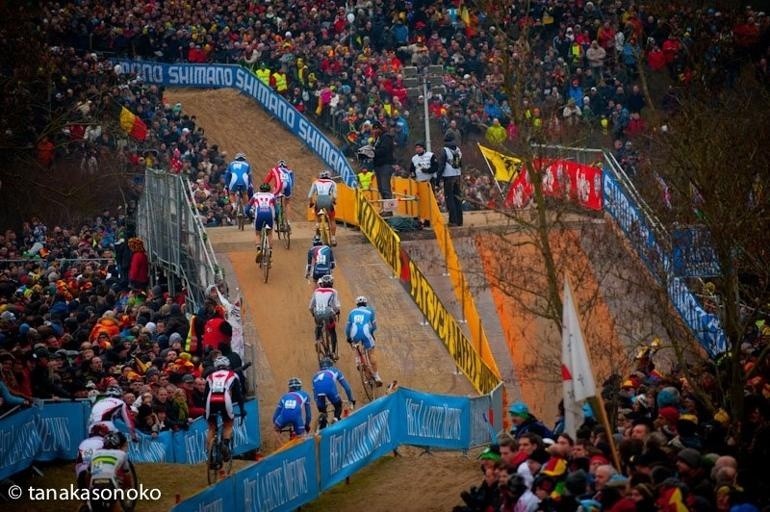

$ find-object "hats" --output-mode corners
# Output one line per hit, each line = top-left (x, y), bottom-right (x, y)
(444, 132), (454, 141)
(661, 407), (679, 422)
(509, 401), (528, 414)
(576, 497), (601, 512)
(260, 182), (270, 191)
(415, 140), (424, 147)
(478, 444), (500, 460)
(531, 449), (549, 465)
(679, 448), (701, 470)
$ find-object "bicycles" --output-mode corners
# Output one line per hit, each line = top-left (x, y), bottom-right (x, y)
(313, 209), (335, 249)
(257, 226), (273, 286)
(115, 456), (138, 511)
(315, 310), (339, 369)
(206, 412), (246, 486)
(270, 396), (356, 439)
(233, 183), (247, 231)
(276, 198), (294, 249)
(354, 341), (378, 403)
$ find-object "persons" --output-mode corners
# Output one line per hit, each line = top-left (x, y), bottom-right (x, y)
(409, 142), (438, 230)
(437, 126), (466, 227)
(303, 233), (337, 281)
(309, 275), (342, 354)
(371, 121), (397, 218)
(307, 168), (337, 247)
(270, 377), (312, 447)
(345, 295), (384, 388)
(75, 421), (110, 486)
(80, 430), (140, 511)
(89, 385), (139, 451)
(198, 356), (247, 470)
(259, 157), (295, 228)
(253, 1), (768, 208)
(627, 206), (769, 512)
(456, 371), (628, 510)
(310, 357), (354, 429)
(0, 2), (253, 427)
(245, 182), (278, 267)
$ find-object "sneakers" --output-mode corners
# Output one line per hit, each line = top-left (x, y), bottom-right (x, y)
(221, 443), (230, 458)
(376, 377), (383, 387)
(330, 240), (337, 246)
(255, 250), (262, 264)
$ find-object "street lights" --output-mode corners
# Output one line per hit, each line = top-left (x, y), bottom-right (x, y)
(401, 64), (445, 156)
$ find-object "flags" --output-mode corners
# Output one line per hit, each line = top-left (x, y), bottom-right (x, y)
(560, 275), (599, 442)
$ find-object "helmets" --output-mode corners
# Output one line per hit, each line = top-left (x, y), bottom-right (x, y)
(320, 357), (333, 367)
(106, 385), (122, 396)
(288, 379), (301, 387)
(313, 236), (321, 245)
(318, 274), (334, 287)
(102, 432), (128, 449)
(319, 169), (332, 179)
(235, 152), (246, 160)
(278, 159), (286, 167)
(355, 295), (366, 304)
(214, 356), (229, 368)
(89, 422), (111, 436)
